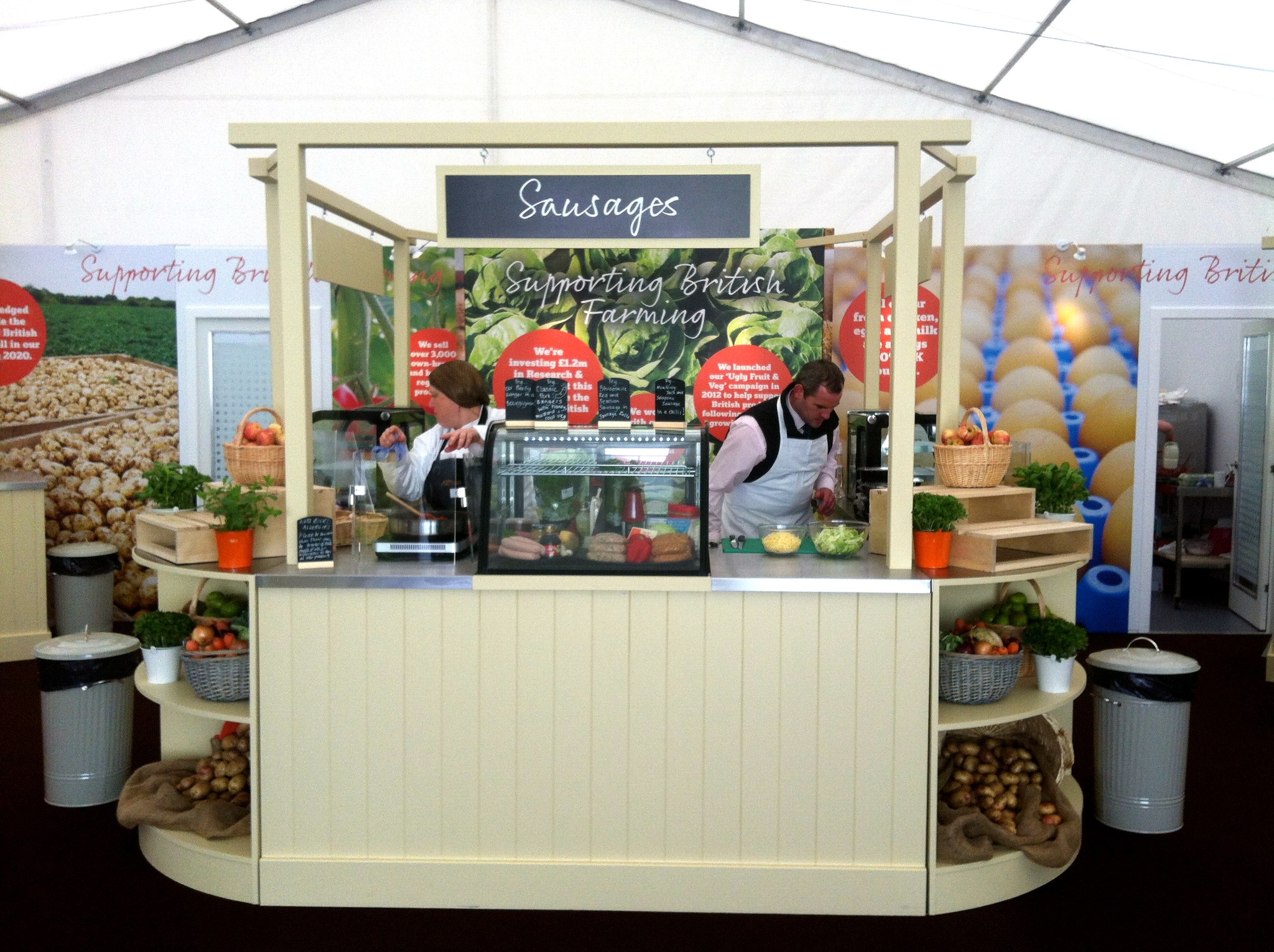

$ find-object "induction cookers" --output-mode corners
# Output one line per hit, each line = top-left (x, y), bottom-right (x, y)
(373, 532), (481, 561)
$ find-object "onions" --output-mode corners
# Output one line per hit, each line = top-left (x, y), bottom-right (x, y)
(955, 642), (973, 655)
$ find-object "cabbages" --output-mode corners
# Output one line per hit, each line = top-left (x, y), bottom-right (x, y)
(812, 523), (865, 555)
(344, 233), (833, 436)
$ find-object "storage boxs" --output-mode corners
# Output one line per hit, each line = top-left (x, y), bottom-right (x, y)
(478, 417), (713, 577)
(846, 408), (937, 524)
(312, 406), (427, 517)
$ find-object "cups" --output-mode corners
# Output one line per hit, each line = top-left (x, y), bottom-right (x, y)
(1214, 470), (1230, 487)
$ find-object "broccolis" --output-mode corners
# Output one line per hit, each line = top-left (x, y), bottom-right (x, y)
(1020, 614), (1089, 663)
(935, 631), (962, 651)
(134, 608), (185, 655)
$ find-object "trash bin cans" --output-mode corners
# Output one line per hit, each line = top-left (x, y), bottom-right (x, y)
(46, 542), (120, 635)
(1085, 633), (1202, 835)
(32, 624), (142, 808)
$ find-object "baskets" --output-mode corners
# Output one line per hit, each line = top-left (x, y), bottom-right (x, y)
(180, 578), (249, 625)
(223, 406), (285, 486)
(938, 712), (1075, 787)
(939, 644), (1024, 705)
(179, 637), (249, 702)
(970, 579), (1046, 677)
(933, 407), (1013, 487)
(336, 509), (388, 547)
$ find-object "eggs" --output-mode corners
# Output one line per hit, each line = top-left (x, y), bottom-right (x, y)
(825, 245), (1132, 577)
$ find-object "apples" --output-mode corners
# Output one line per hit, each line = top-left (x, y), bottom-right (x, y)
(235, 422), (284, 445)
(941, 423), (1010, 445)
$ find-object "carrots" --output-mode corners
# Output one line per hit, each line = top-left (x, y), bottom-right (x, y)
(185, 620), (233, 660)
(999, 641), (1019, 655)
(953, 617), (983, 637)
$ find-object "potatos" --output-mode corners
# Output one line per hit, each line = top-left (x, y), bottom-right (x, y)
(176, 724), (249, 806)
(1, 353), (179, 618)
(943, 739), (1060, 835)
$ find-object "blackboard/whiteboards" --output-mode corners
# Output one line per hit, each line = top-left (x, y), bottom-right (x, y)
(653, 378), (688, 431)
(597, 378), (631, 429)
(533, 378), (569, 429)
(295, 513), (335, 571)
(504, 377), (535, 429)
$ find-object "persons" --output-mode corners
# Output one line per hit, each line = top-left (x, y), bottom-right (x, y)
(371, 360), (508, 503)
(704, 359), (845, 548)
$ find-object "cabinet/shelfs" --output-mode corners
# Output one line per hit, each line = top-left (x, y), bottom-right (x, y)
(137, 481), (336, 564)
(1156, 479), (1234, 610)
(868, 484), (1094, 573)
(0, 469), (52, 667)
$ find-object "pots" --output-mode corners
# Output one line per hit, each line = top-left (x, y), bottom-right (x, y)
(386, 511), (474, 538)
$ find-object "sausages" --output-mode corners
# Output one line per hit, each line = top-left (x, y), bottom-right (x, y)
(499, 535), (543, 560)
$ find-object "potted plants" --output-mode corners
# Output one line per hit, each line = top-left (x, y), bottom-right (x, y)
(133, 611), (194, 685)
(1021, 615), (1087, 693)
(913, 491), (968, 570)
(1010, 461), (1089, 523)
(191, 473), (283, 569)
(134, 459), (212, 514)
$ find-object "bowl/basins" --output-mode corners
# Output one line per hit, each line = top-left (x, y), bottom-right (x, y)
(758, 520), (871, 557)
(1183, 539), (1215, 555)
